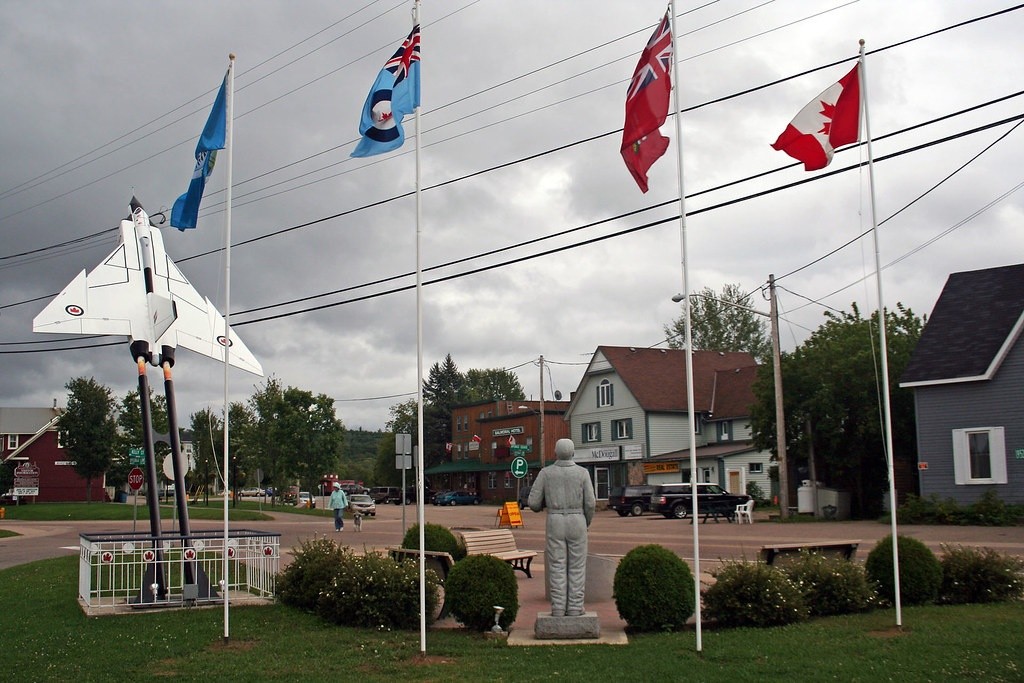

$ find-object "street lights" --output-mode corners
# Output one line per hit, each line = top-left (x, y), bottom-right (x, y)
(518, 405), (545, 467)
(672, 293), (789, 521)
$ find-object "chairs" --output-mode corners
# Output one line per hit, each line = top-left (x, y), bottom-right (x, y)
(735, 500), (754, 524)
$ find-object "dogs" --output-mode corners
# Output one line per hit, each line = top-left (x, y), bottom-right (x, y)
(353, 512), (362, 532)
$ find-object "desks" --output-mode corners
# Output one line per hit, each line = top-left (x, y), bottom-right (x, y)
(690, 500), (739, 525)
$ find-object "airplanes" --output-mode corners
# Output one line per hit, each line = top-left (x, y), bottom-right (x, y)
(32, 185), (264, 381)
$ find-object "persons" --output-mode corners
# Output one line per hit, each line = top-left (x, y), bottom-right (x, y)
(527, 439), (596, 618)
(328, 483), (349, 531)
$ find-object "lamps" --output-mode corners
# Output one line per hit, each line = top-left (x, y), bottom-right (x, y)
(492, 605), (506, 630)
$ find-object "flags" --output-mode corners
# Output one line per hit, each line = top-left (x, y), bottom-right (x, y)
(471, 435), (481, 444)
(620, 6), (676, 195)
(351, 25), (422, 157)
(770, 60), (865, 170)
(446, 443), (452, 451)
(170, 65), (232, 233)
(507, 435), (516, 447)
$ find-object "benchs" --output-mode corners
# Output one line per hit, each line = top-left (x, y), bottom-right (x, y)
(385, 547), (457, 584)
(459, 529), (537, 579)
(760, 539), (861, 566)
(697, 507), (736, 513)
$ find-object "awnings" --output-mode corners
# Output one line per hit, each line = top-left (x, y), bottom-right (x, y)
(424, 459), (555, 474)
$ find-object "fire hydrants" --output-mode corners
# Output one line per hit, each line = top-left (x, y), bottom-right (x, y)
(0, 508), (6, 519)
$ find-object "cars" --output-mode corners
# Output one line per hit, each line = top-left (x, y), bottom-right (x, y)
(294, 492), (315, 508)
(348, 494), (375, 516)
(342, 483), (370, 494)
(431, 490), (483, 506)
(239, 487), (265, 497)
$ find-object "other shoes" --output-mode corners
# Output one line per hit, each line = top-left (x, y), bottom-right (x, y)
(340, 527), (343, 531)
(336, 528), (340, 532)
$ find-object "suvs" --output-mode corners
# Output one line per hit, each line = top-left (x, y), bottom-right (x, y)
(283, 485), (297, 502)
(373, 487), (400, 505)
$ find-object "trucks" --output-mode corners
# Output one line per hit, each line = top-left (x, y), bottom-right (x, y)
(319, 478), (354, 496)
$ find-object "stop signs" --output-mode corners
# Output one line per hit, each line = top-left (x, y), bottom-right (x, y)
(128, 468), (144, 490)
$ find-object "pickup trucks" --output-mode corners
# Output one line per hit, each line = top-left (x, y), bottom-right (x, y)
(608, 484), (658, 516)
(401, 486), (431, 504)
(650, 483), (748, 519)
